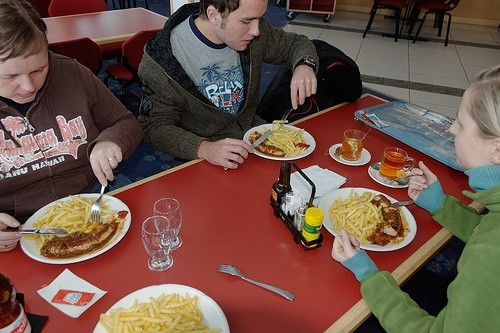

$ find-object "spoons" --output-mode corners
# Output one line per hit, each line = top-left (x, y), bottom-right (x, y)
(370, 162), (408, 185)
(352, 128), (371, 158)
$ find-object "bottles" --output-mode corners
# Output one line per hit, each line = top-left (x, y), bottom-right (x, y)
(271, 162), (292, 207)
(300, 207), (324, 248)
(294, 207), (306, 231)
(282, 191), (294, 215)
(0, 271), (32, 333)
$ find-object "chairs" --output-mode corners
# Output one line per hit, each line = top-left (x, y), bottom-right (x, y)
(30, 0), (162, 110)
(362, 0), (461, 47)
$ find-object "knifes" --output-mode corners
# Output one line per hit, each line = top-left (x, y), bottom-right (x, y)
(391, 200), (413, 207)
(4, 229), (68, 234)
(223, 129), (271, 170)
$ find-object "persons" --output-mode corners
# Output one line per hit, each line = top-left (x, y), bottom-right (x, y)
(136, 0), (320, 170)
(330, 67), (500, 333)
(0, 0), (141, 253)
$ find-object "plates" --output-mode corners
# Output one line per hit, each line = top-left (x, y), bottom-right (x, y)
(242, 123), (316, 160)
(19, 193), (132, 264)
(329, 143), (371, 166)
(368, 162), (414, 188)
(318, 188), (417, 251)
(92, 284), (231, 333)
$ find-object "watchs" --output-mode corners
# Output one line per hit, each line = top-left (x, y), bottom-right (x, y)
(295, 56), (316, 72)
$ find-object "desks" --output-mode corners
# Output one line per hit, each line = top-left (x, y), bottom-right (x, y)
(0, 94), (486, 333)
(41, 7), (171, 57)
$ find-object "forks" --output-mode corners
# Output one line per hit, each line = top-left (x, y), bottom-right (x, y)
(282, 103), (299, 123)
(392, 173), (424, 185)
(91, 177), (107, 221)
(215, 264), (295, 301)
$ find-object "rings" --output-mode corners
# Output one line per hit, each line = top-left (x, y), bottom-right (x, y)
(5, 245), (8, 249)
(110, 157), (116, 161)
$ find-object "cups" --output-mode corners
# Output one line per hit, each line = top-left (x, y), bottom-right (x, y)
(341, 129), (366, 161)
(379, 146), (416, 180)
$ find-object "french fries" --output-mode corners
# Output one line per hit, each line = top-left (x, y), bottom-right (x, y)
(24, 194), (120, 250)
(328, 188), (384, 242)
(100, 291), (222, 333)
(261, 120), (307, 157)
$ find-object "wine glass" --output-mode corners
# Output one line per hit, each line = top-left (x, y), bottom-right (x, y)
(153, 198), (182, 251)
(141, 215), (173, 271)
